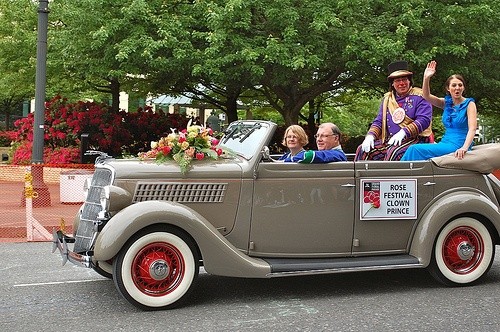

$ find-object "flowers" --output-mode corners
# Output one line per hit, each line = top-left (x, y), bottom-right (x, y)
(139, 119), (236, 174)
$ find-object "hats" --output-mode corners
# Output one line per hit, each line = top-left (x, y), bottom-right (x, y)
(388, 61), (413, 79)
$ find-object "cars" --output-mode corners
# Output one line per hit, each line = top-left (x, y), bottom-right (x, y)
(52, 120), (500, 311)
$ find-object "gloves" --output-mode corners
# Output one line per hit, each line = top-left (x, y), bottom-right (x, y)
(362, 135), (375, 152)
(388, 129), (407, 146)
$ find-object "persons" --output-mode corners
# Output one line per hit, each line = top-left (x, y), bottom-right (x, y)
(285, 123), (348, 163)
(353, 60), (436, 161)
(274, 125), (309, 162)
(399, 61), (477, 162)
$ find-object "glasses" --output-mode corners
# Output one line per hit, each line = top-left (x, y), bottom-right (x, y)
(314, 134), (332, 139)
